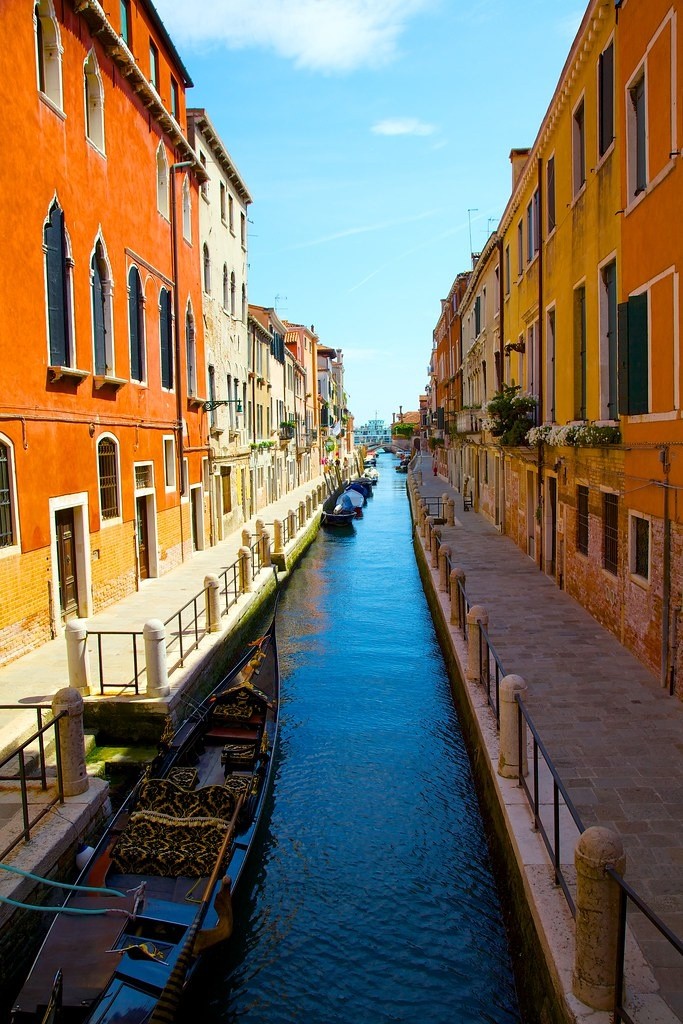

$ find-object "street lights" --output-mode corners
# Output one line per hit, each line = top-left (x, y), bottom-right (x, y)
(200, 398), (244, 546)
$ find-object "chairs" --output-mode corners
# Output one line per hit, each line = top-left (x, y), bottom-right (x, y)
(221, 744), (258, 777)
(464, 491), (473, 508)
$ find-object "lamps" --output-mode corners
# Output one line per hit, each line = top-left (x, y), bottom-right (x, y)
(203, 398), (243, 416)
(433, 412), (455, 422)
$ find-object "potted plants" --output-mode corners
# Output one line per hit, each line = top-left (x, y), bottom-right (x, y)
(280, 422), (296, 446)
(268, 441), (276, 450)
(249, 443), (258, 454)
(259, 442), (269, 451)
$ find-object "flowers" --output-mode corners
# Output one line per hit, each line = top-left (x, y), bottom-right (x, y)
(482, 392), (621, 448)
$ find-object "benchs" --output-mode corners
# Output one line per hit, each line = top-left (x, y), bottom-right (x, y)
(111, 779), (235, 876)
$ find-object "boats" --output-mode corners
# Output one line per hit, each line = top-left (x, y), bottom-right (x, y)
(6, 590), (282, 1024)
(320, 452), (379, 526)
(395, 450), (411, 473)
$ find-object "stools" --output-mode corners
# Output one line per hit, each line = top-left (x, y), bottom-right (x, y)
(223, 774), (253, 803)
(166, 766), (198, 791)
